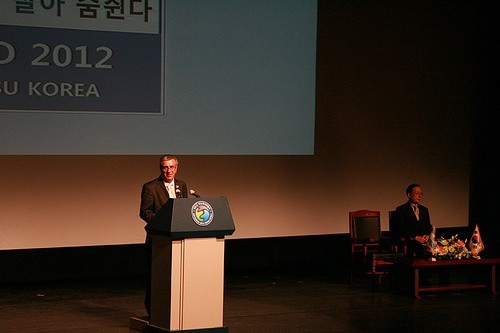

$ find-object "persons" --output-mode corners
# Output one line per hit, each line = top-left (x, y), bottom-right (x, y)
(139, 155), (188, 317)
(390, 184), (449, 297)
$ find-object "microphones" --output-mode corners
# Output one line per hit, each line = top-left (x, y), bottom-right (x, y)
(189, 190), (200, 198)
(176, 189), (183, 198)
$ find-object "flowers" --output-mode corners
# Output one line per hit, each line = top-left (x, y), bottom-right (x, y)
(416, 234), (477, 260)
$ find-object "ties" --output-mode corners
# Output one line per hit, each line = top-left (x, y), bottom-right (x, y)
(168, 185), (175, 198)
(415, 206), (420, 221)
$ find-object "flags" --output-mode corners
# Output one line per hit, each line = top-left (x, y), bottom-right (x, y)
(469, 224), (485, 257)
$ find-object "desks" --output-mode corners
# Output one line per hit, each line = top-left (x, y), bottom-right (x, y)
(400, 258), (500, 301)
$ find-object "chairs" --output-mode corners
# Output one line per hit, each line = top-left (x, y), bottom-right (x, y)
(349, 210), (408, 293)
